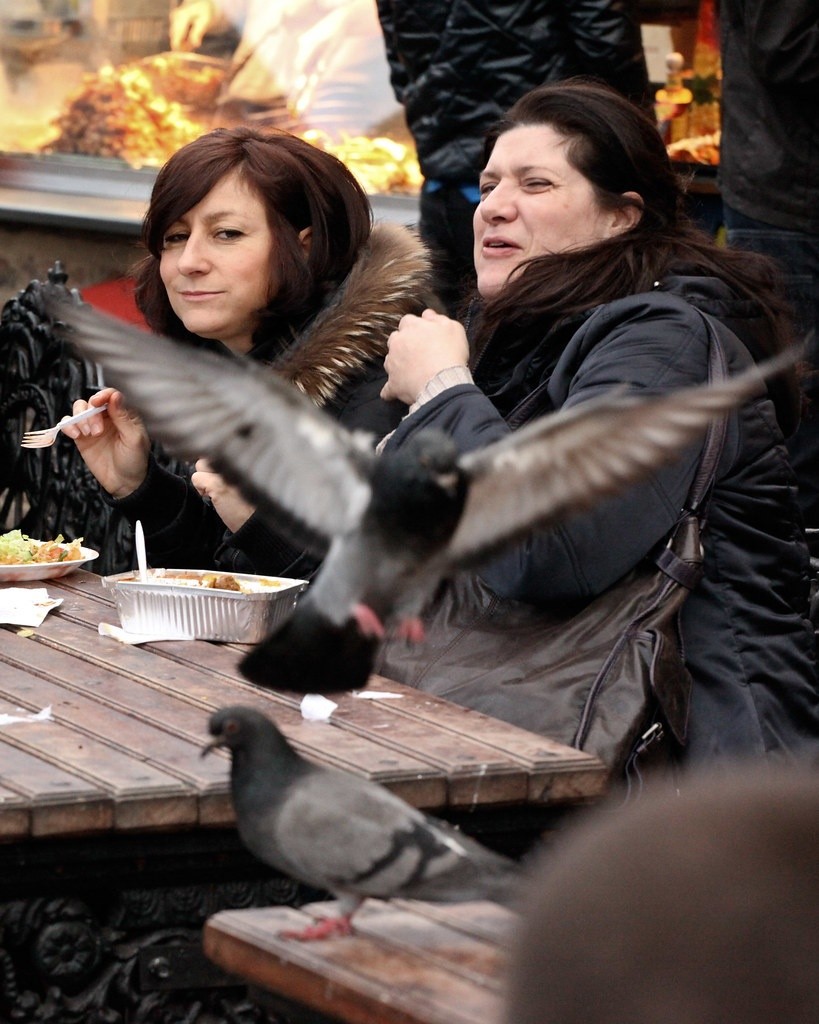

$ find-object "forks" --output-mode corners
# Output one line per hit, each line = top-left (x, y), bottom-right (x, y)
(20, 404), (108, 448)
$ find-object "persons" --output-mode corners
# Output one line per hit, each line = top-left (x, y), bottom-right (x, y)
(358, 82), (819, 786)
(62, 126), (455, 602)
(371, 1), (662, 336)
(708, 1), (819, 328)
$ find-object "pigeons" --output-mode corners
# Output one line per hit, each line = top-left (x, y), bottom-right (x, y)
(200, 706), (524, 942)
(53, 296), (811, 694)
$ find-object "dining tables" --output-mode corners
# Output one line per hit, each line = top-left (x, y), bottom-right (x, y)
(203, 889), (525, 1024)
(0, 569), (606, 1024)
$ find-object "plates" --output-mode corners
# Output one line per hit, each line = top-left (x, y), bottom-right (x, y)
(0, 542), (99, 581)
